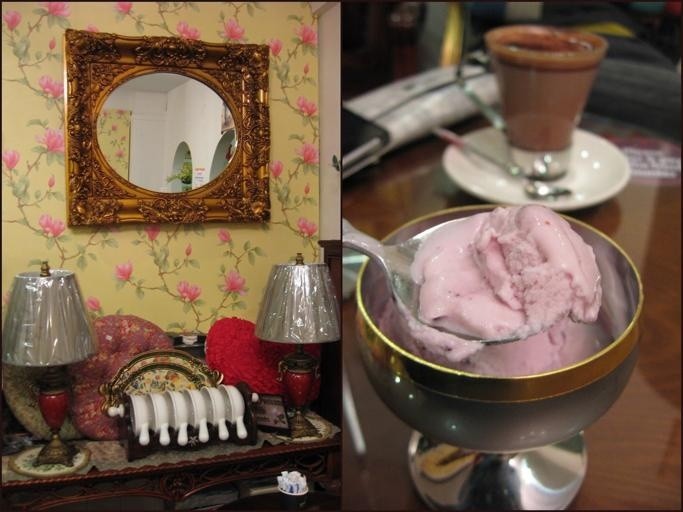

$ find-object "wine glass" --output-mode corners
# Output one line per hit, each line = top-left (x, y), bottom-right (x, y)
(355, 204), (644, 512)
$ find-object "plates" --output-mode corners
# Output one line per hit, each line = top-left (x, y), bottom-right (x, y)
(98, 348), (224, 417)
(442, 125), (630, 211)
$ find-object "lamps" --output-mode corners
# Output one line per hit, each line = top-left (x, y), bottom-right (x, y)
(1, 261), (97, 468)
(254, 253), (341, 438)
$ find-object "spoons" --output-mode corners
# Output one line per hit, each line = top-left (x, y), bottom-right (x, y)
(457, 75), (571, 200)
(340, 216), (520, 346)
(431, 124), (566, 180)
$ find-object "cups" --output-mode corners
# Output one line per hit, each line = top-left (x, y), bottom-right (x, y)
(488, 26), (607, 152)
(200, 386), (229, 440)
(128, 394), (149, 446)
(277, 486), (308, 512)
(145, 393), (171, 445)
(183, 390), (210, 442)
(218, 384), (248, 439)
(164, 391), (189, 446)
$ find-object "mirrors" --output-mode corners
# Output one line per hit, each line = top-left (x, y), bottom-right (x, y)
(63, 27), (272, 227)
